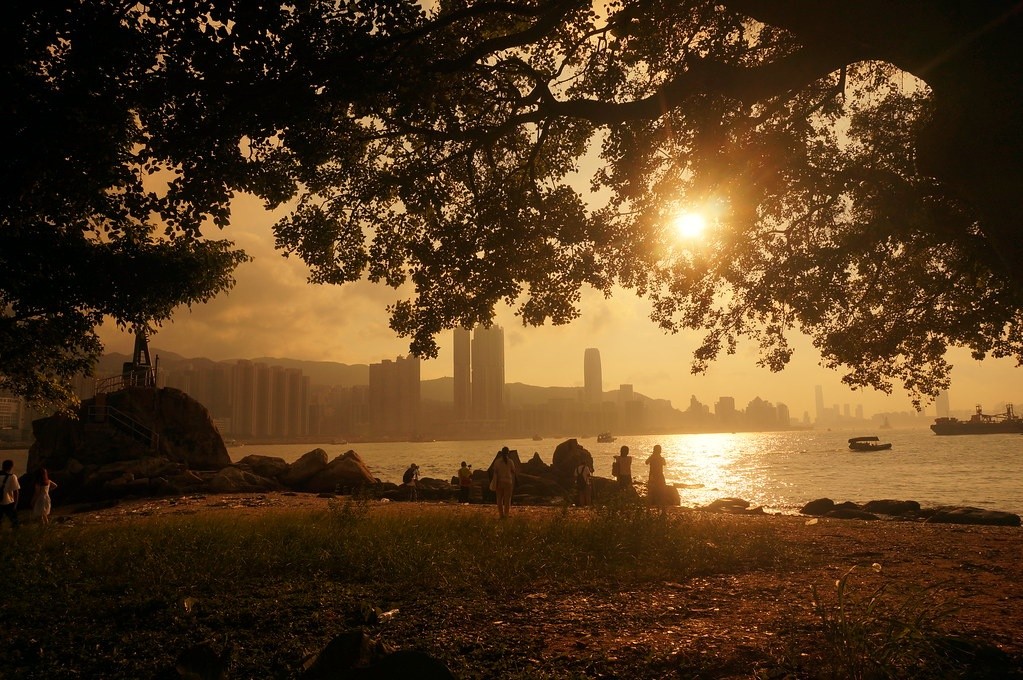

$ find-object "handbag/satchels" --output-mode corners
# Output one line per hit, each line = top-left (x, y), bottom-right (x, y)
(612, 456), (618, 477)
(490, 472), (497, 491)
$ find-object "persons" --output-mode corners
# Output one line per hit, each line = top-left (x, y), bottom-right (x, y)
(458, 461), (473, 505)
(30, 468), (58, 525)
(405, 463), (421, 502)
(494, 446), (515, 518)
(615, 445), (639, 510)
(575, 460), (592, 507)
(0, 460), (21, 525)
(645, 445), (666, 506)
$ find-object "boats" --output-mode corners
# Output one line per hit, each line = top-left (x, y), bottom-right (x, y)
(930, 402), (1023, 436)
(597, 431), (618, 444)
(848, 435), (893, 452)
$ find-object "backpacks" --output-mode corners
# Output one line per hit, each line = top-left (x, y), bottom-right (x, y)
(403, 468), (415, 483)
(576, 466), (589, 488)
